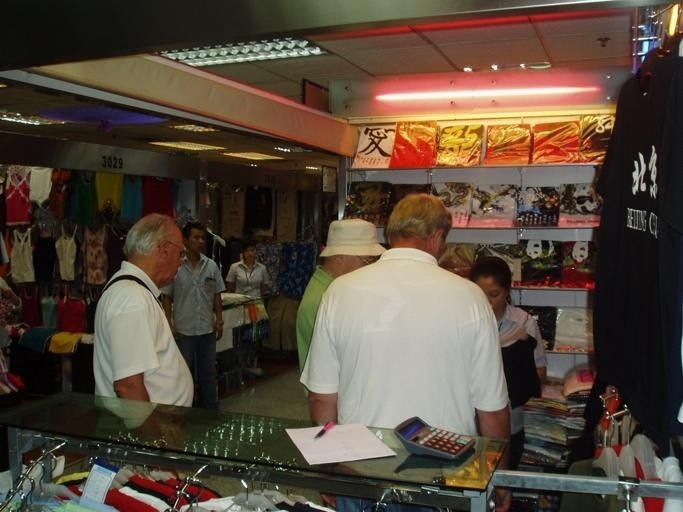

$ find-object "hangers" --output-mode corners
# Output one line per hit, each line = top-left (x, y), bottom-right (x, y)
(593, 406), (675, 482)
(5, 448), (295, 512)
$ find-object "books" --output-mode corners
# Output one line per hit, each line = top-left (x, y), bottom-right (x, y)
(284, 425), (397, 466)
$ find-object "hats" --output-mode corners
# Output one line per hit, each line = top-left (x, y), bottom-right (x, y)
(318, 217), (388, 258)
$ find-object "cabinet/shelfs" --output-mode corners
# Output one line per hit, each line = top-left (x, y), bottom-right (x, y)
(345, 164), (600, 381)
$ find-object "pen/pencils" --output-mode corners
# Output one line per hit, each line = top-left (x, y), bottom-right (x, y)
(315, 419), (336, 438)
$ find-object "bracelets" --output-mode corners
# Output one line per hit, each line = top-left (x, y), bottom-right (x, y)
(216, 321), (224, 325)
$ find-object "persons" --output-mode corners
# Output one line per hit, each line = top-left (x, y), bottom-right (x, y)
(305, 192), (517, 511)
(468, 254), (551, 461)
(294, 218), (385, 402)
(226, 241), (270, 378)
(92, 208), (196, 462)
(162, 217), (225, 410)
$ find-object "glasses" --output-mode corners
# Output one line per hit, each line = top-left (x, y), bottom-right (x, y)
(157, 239), (188, 259)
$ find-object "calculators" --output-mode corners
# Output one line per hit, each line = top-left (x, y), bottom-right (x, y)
(393, 417), (475, 460)
